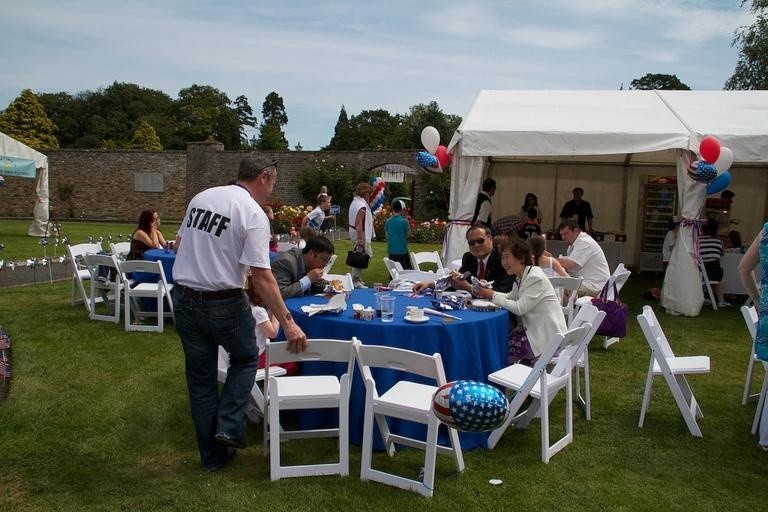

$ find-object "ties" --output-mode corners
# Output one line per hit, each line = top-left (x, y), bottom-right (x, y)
(477, 260), (486, 280)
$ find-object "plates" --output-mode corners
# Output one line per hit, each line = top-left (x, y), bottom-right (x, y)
(404, 316), (431, 323)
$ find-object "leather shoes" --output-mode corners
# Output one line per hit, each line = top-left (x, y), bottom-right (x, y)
(170, 285), (246, 299)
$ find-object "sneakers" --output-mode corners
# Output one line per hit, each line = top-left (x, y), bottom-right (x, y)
(202, 448), (237, 471)
(214, 431), (248, 449)
(718, 302), (731, 308)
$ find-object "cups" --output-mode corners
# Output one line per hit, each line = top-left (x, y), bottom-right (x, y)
(433, 289), (443, 300)
(406, 308), (425, 319)
(375, 292), (391, 319)
(406, 307), (426, 320)
(380, 295), (396, 322)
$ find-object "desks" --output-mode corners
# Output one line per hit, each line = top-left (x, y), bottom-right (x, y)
(546, 240), (624, 277)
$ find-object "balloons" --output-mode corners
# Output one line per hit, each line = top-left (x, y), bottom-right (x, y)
(415, 125), (450, 177)
(368, 176), (384, 214)
(686, 136), (735, 193)
(430, 379), (509, 432)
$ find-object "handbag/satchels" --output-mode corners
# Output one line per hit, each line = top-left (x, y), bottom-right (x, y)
(589, 278), (629, 338)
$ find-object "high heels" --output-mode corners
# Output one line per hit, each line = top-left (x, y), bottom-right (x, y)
(345, 243), (370, 269)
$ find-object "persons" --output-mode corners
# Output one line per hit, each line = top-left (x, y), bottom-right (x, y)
(383, 200), (411, 282)
(125, 208), (176, 323)
(171, 151), (309, 474)
(348, 183), (376, 289)
(229, 179), (337, 443)
(413, 177), (612, 421)
(662, 190), (742, 308)
(736, 221), (767, 454)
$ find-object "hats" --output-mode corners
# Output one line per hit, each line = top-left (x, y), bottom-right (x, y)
(392, 200), (405, 211)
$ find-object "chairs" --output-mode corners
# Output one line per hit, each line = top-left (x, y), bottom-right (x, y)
(353, 340), (466, 498)
(486, 321), (592, 465)
(538, 303), (607, 421)
(636, 305), (711, 439)
(546, 276), (584, 327)
(264, 336), (358, 482)
(383, 251), (462, 292)
(740, 305), (768, 435)
(217, 345), (290, 442)
(599, 264), (631, 304)
(299, 239), (354, 292)
(698, 257), (720, 311)
(66, 242), (180, 333)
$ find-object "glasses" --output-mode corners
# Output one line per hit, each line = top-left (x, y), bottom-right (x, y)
(255, 158), (279, 176)
(468, 236), (491, 246)
(313, 252), (332, 266)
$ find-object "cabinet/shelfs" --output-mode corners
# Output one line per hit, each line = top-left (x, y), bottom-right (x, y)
(637, 174), (678, 275)
